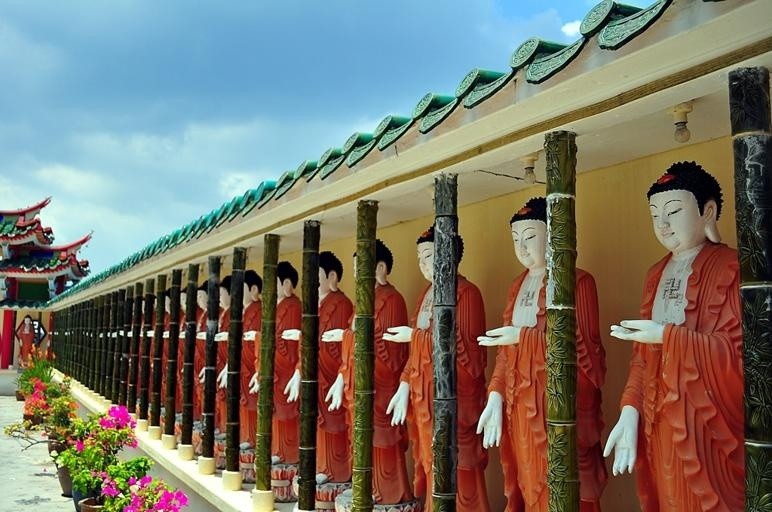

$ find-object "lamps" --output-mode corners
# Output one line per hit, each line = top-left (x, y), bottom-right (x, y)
(666, 101), (695, 143)
(520, 151), (541, 191)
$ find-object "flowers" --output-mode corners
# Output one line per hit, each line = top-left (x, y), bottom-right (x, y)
(3, 359), (192, 511)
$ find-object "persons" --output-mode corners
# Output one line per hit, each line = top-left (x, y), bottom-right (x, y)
(18, 316), (35, 363)
(283, 251), (353, 484)
(128, 262), (301, 465)
(324, 239), (409, 504)
(476, 195), (607, 512)
(381, 219), (490, 512)
(601, 160), (746, 512)
